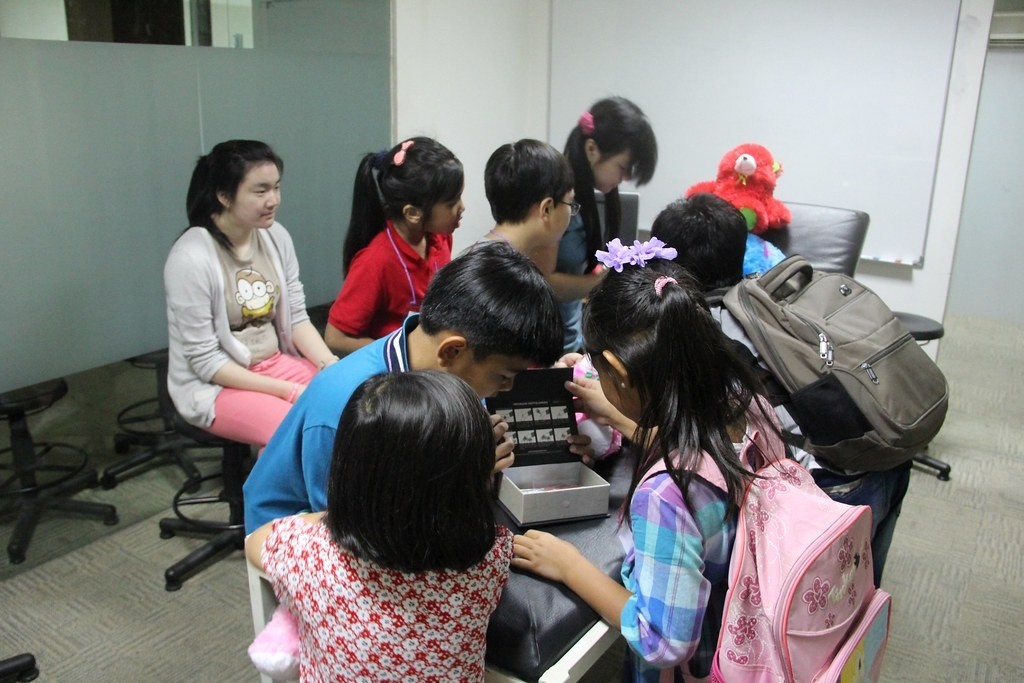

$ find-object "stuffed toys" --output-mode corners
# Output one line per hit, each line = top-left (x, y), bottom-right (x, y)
(684, 143), (792, 235)
(735, 231), (787, 280)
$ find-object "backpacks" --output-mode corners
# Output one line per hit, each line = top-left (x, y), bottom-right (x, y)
(631, 393), (892, 683)
(703, 254), (948, 475)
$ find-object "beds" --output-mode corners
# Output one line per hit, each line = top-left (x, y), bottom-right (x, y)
(247, 204), (869, 683)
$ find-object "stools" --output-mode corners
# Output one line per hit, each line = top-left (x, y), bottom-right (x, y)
(0, 311), (949, 683)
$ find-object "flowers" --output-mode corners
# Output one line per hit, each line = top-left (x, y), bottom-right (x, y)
(595, 234), (678, 272)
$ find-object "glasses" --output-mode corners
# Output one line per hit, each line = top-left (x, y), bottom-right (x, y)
(560, 200), (581, 216)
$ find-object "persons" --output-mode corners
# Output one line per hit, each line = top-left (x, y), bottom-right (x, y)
(527, 96), (658, 355)
(163, 139), (340, 460)
(244, 367), (513, 683)
(324, 135), (466, 360)
(510, 236), (800, 683)
(649, 192), (914, 683)
(241, 239), (565, 537)
(473, 139), (582, 256)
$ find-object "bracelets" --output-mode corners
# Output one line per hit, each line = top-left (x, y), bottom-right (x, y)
(288, 382), (299, 403)
(317, 354), (340, 372)
